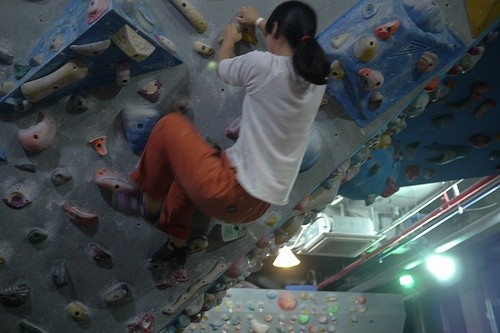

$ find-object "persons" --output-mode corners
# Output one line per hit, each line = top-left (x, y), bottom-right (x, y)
(117, 0), (330, 261)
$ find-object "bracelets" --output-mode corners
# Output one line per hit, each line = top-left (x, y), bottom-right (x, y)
(254, 17), (265, 27)
